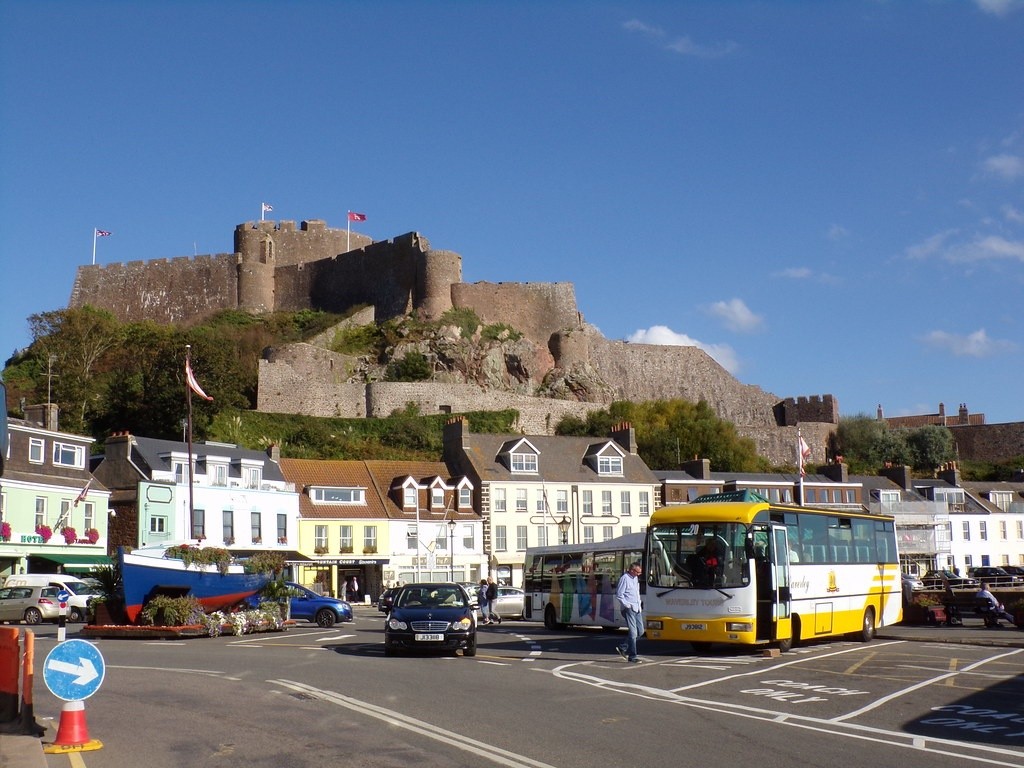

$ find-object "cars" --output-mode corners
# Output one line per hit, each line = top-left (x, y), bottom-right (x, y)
(243, 581), (353, 629)
(378, 581), (524, 624)
(965, 564), (1024, 588)
(901, 572), (924, 591)
(919, 569), (981, 590)
(382, 582), (480, 657)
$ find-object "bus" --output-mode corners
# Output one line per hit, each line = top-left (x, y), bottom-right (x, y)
(522, 532), (734, 638)
(637, 487), (903, 655)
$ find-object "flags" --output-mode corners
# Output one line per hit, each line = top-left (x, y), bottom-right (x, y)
(350, 212), (367, 222)
(264, 204), (273, 211)
(97, 230), (112, 236)
(800, 437), (811, 476)
(185, 356), (213, 400)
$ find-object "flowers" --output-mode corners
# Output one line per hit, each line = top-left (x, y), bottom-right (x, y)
(87, 529), (99, 543)
(1, 521), (11, 538)
(36, 525), (52, 541)
(62, 526), (77, 544)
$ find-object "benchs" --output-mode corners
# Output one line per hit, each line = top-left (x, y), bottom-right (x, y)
(943, 597), (1005, 627)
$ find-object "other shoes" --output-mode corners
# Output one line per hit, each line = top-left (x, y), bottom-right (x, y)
(489, 622), (493, 624)
(481, 622), (490, 625)
(629, 658), (642, 663)
(498, 617), (502, 624)
(616, 646), (627, 661)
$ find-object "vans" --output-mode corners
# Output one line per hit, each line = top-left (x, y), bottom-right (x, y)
(1, 573), (107, 623)
(0, 585), (72, 625)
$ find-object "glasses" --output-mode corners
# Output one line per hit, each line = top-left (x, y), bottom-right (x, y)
(633, 569), (642, 576)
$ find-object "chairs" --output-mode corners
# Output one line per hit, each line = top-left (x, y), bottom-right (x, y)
(792, 538), (889, 563)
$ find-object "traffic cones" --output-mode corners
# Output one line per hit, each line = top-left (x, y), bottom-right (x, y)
(43, 700), (105, 755)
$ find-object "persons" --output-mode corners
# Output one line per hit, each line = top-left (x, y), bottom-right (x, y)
(478, 579), (489, 624)
(340, 581), (347, 601)
(486, 576), (502, 624)
(976, 581), (1013, 623)
(349, 577), (359, 602)
(615, 562), (645, 664)
(788, 540), (799, 562)
